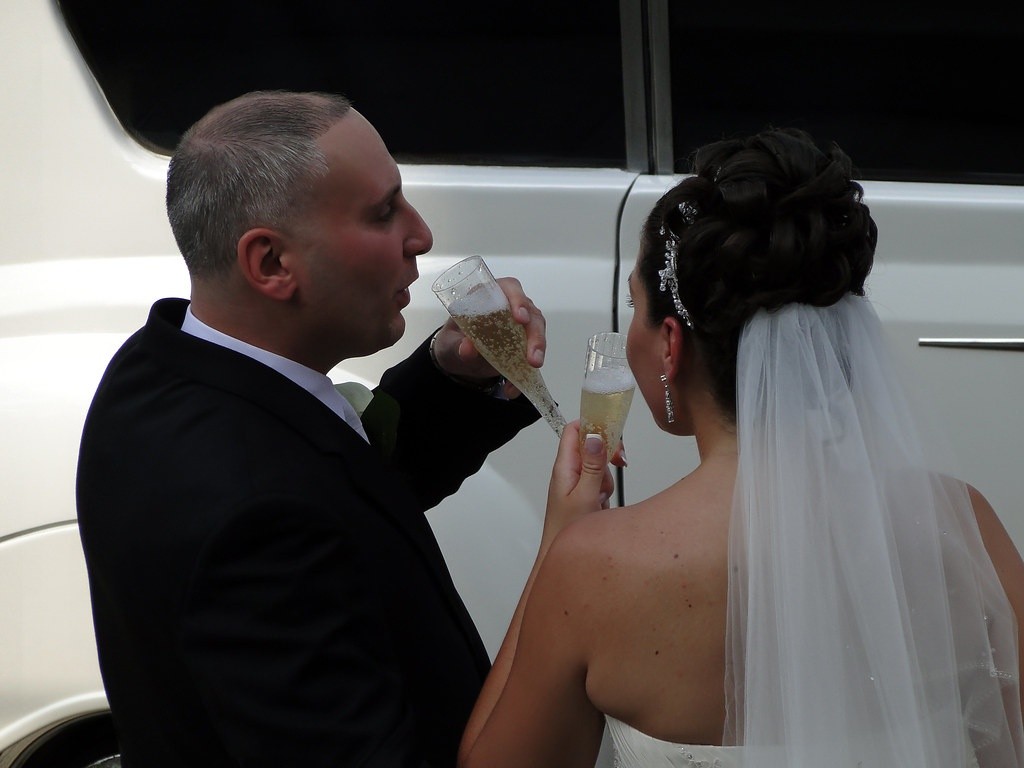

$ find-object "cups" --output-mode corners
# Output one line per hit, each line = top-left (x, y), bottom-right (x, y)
(578, 332), (638, 464)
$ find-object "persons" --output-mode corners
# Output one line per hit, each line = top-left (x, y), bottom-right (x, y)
(75, 90), (543, 768)
(456, 125), (1024, 768)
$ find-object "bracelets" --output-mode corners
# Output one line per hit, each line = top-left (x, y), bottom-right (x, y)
(429, 328), (447, 369)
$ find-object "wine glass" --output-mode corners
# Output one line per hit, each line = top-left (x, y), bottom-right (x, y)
(432, 255), (569, 441)
